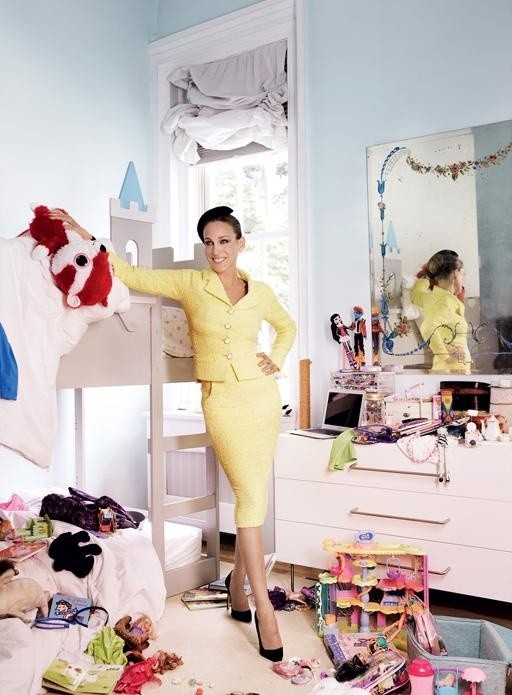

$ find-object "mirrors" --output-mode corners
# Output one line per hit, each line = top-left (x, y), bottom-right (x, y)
(365, 117), (512, 376)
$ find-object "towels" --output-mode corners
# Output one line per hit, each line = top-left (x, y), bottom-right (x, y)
(83, 626), (128, 667)
(328, 428), (357, 471)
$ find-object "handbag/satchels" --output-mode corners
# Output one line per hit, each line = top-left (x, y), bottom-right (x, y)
(38, 486), (145, 531)
(393, 417), (443, 464)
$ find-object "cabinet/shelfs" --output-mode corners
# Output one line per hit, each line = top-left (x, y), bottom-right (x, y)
(275, 432), (512, 619)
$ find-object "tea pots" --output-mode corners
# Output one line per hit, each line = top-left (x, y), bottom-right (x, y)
(480, 414), (501, 443)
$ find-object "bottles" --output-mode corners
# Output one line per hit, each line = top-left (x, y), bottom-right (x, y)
(406, 660), (435, 695)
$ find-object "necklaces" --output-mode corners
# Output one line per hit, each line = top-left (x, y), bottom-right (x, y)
(407, 432), (439, 466)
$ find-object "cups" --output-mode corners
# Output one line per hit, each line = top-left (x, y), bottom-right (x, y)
(464, 430), (479, 447)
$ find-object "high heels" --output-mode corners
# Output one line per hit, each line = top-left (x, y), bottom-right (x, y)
(224, 570), (252, 624)
(254, 611), (283, 662)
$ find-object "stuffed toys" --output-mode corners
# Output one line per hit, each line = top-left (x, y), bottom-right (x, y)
(0, 560), (49, 625)
(113, 610), (184, 695)
(14, 202), (113, 309)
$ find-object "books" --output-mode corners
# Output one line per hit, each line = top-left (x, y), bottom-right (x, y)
(0, 542), (50, 563)
(48, 592), (94, 626)
(390, 416), (450, 439)
(43, 647), (127, 695)
(178, 551), (276, 613)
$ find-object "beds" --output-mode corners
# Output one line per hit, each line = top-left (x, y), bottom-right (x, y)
(0, 201), (220, 599)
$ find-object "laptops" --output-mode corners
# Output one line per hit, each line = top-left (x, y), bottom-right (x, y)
(289, 389), (365, 439)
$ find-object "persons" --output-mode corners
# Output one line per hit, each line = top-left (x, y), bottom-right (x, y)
(330, 313), (360, 370)
(371, 305), (384, 367)
(344, 306), (367, 368)
(45, 203), (303, 664)
(413, 248), (478, 376)
